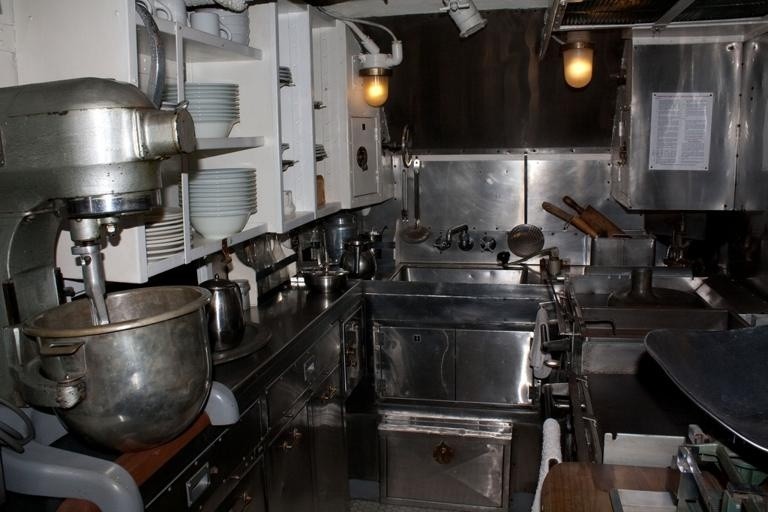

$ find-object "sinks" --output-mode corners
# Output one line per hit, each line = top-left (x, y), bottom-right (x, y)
(385, 260), (528, 285)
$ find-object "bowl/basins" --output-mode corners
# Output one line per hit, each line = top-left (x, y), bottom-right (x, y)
(163, 82), (241, 139)
(178, 168), (258, 239)
(198, 8), (250, 45)
(304, 272), (343, 294)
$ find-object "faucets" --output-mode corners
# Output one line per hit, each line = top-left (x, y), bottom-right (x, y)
(432, 221), (474, 251)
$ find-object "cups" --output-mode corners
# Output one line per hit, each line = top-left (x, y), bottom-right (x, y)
(146, 2), (196, 27)
(190, 13), (232, 41)
(283, 190), (296, 218)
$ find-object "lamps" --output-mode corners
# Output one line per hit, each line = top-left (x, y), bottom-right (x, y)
(558, 22), (599, 90)
(349, 25), (407, 112)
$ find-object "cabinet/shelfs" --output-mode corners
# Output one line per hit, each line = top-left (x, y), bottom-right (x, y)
(368, 317), (556, 508)
(143, 324), (357, 511)
(604, 25), (766, 218)
(10, 2), (387, 281)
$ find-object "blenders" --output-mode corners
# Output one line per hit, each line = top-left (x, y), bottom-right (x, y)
(1, 78), (241, 510)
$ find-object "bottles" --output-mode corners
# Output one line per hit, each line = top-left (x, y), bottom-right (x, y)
(271, 233), (290, 281)
(261, 233), (281, 287)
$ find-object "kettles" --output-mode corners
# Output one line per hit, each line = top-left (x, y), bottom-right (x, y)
(199, 273), (244, 352)
(341, 238), (378, 280)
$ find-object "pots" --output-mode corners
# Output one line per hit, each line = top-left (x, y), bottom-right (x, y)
(21, 285), (214, 453)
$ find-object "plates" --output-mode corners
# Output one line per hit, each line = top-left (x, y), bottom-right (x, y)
(146, 205), (193, 263)
(278, 65), (291, 83)
(315, 144), (327, 159)
